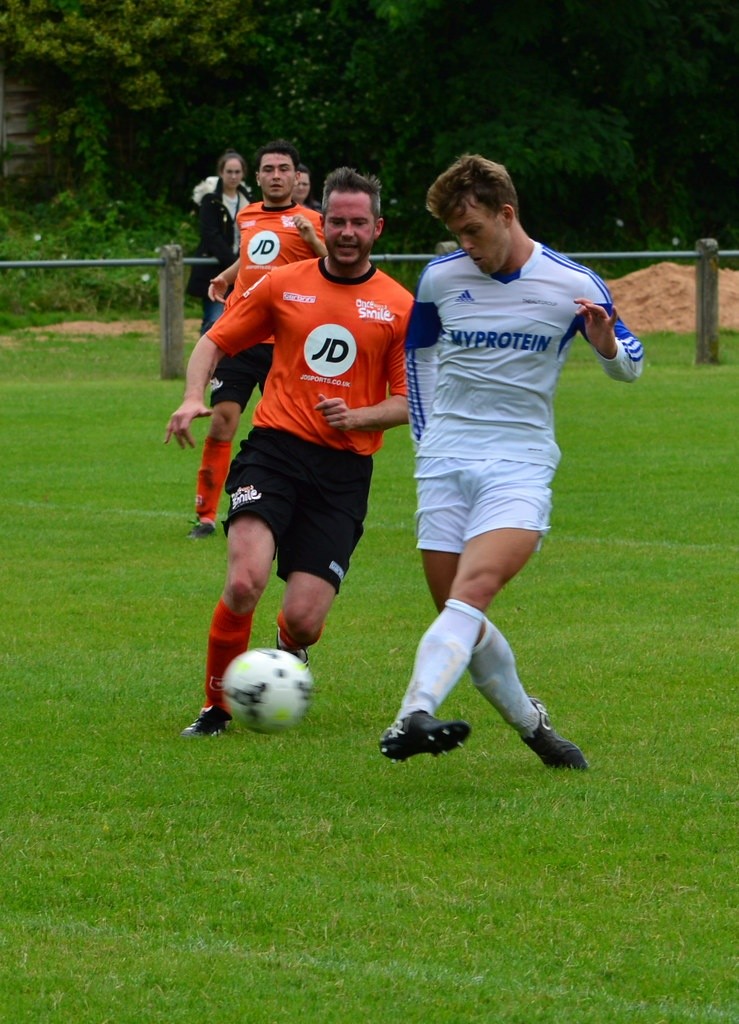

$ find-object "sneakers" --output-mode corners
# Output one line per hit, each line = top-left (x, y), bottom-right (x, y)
(375, 710), (472, 761)
(526, 699), (607, 768)
(181, 705), (242, 740)
(273, 631), (309, 674)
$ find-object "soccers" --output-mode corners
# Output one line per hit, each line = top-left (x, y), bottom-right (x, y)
(223, 647), (314, 735)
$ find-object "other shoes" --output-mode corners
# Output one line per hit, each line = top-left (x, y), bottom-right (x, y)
(187, 519), (216, 538)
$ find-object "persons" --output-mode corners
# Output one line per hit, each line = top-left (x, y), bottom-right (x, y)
(163, 167), (415, 739)
(185, 152), (252, 337)
(186, 139), (328, 539)
(377, 153), (644, 772)
(291, 165), (321, 212)
